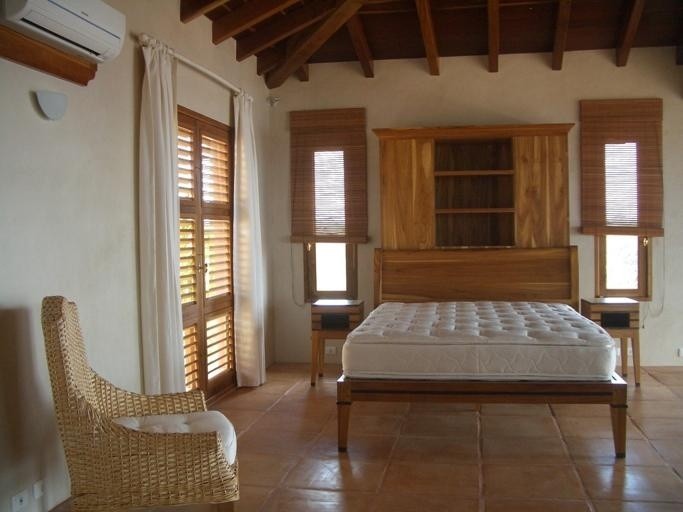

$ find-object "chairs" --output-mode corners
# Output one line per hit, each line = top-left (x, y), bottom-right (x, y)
(40, 296), (242, 512)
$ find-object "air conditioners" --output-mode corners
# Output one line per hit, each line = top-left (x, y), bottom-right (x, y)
(0, 0), (127, 63)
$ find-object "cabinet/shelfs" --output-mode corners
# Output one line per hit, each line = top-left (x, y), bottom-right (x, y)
(514, 123), (575, 249)
(435, 124), (515, 249)
(372, 127), (435, 248)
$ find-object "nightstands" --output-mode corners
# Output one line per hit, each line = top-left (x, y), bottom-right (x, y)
(311, 299), (364, 386)
(581, 297), (641, 385)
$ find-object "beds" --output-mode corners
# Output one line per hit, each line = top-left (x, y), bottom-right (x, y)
(336, 249), (628, 459)
